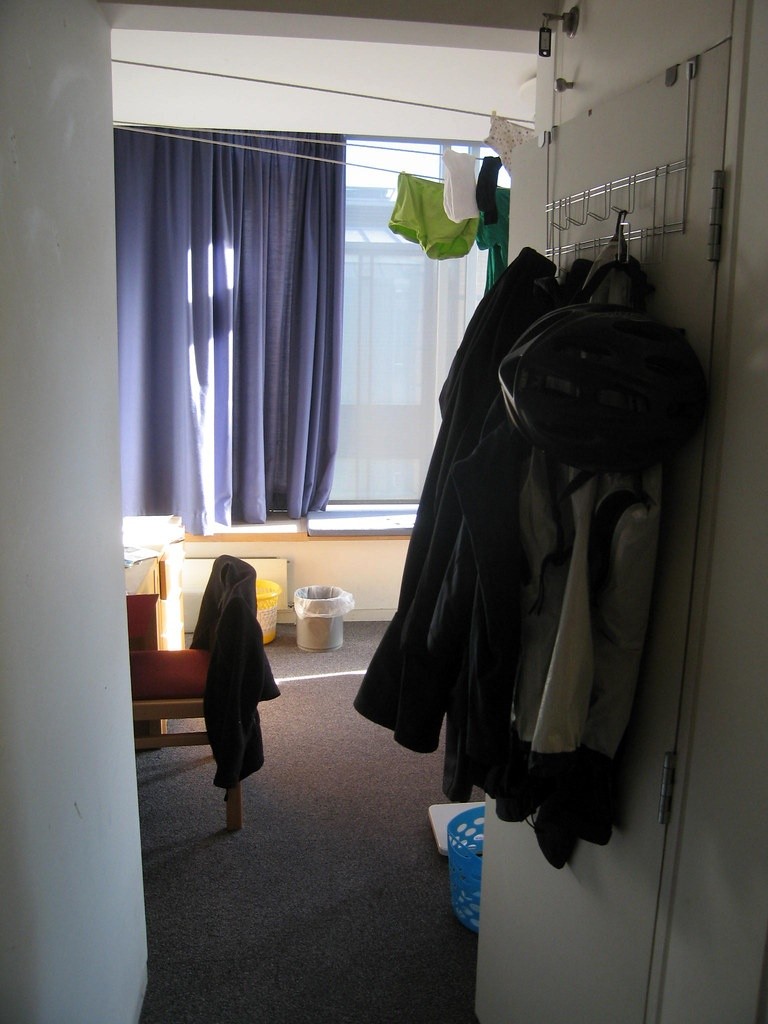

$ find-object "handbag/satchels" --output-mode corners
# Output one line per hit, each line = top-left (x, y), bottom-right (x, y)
(498, 261), (708, 474)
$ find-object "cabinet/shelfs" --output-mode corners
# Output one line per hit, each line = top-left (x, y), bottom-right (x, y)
(123, 517), (185, 751)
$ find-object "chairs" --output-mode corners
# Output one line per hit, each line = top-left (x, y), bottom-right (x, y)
(130, 554), (281, 833)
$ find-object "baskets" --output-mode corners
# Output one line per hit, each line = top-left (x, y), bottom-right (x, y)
(448, 806), (485, 932)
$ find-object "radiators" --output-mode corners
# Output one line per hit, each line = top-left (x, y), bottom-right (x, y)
(184, 557), (295, 613)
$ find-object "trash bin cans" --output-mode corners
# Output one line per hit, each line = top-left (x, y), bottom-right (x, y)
(255, 579), (282, 645)
(294, 585), (344, 654)
